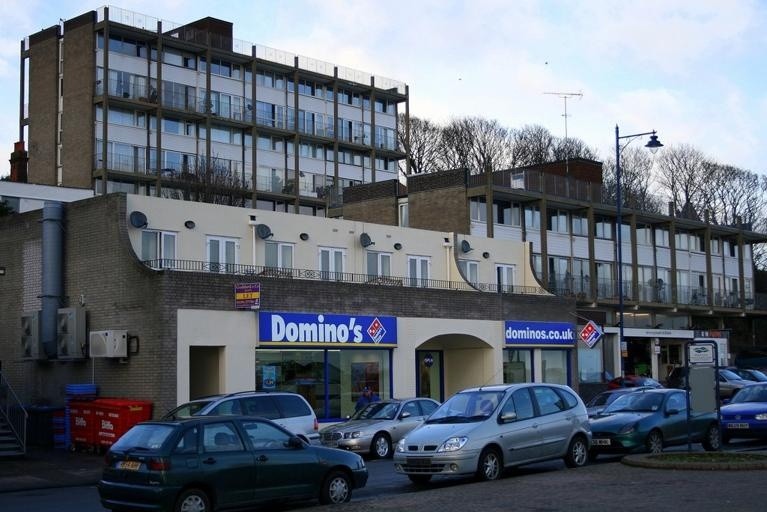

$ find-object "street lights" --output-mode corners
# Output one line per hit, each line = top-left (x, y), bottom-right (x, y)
(614, 125), (663, 369)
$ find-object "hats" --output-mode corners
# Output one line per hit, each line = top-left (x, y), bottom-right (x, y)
(363, 387), (371, 392)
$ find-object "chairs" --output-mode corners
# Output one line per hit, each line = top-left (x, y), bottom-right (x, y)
(478, 400), (492, 415)
(193, 431), (231, 452)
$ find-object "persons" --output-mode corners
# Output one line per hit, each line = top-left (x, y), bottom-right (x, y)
(355, 385), (381, 412)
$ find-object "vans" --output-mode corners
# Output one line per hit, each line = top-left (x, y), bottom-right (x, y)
(156, 389), (320, 446)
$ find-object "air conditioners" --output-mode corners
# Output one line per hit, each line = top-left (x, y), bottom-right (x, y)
(19, 306), (139, 361)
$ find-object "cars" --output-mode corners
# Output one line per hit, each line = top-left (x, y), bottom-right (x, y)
(91, 415), (368, 512)
(392, 383), (592, 483)
(585, 366), (766, 455)
(318, 397), (442, 458)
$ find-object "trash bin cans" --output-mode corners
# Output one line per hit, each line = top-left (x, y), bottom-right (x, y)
(27, 407), (55, 453)
